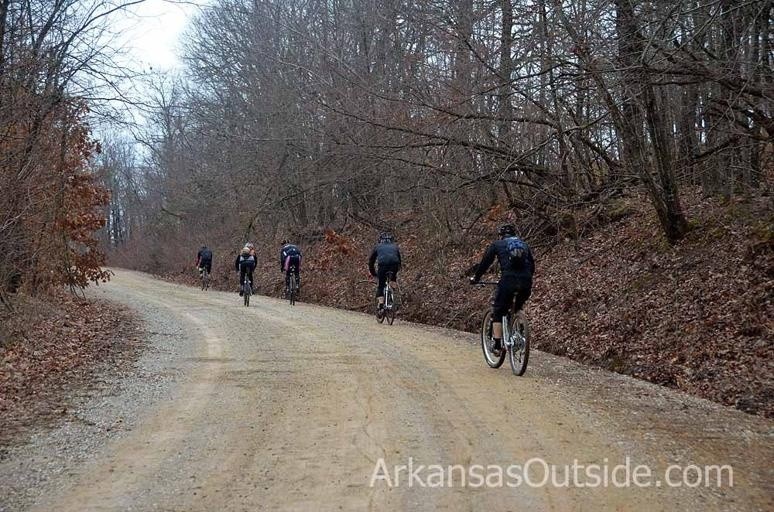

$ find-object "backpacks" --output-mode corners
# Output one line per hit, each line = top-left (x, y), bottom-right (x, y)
(504, 238), (528, 270)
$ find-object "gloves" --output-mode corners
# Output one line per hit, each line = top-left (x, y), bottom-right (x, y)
(470, 276), (478, 283)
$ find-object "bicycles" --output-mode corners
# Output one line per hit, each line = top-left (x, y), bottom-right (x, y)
(236, 269), (255, 307)
(195, 263), (211, 292)
(374, 270), (400, 326)
(468, 277), (539, 377)
(280, 266), (300, 306)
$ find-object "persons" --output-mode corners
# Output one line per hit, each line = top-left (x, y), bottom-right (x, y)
(196, 244), (213, 281)
(235, 242), (257, 297)
(279, 238), (303, 292)
(368, 233), (401, 311)
(469, 225), (535, 355)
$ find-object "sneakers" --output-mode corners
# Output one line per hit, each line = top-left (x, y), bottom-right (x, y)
(490, 343), (501, 356)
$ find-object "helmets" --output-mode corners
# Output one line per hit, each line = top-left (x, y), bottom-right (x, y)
(499, 225), (515, 236)
(379, 233), (392, 243)
(241, 247), (250, 254)
(245, 242), (253, 250)
(282, 239), (288, 244)
